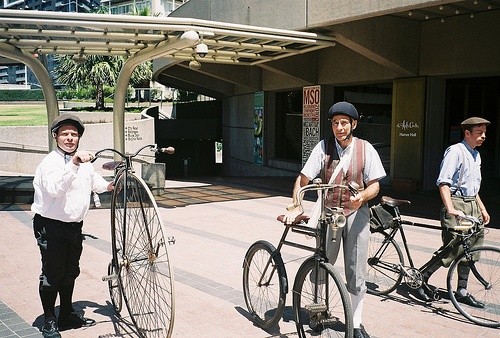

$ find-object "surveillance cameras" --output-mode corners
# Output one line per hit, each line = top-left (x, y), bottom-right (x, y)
(196, 44), (209, 58)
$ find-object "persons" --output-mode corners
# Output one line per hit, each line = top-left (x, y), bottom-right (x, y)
(31, 114), (123, 338)
(408, 117), (491, 308)
(282, 102), (386, 338)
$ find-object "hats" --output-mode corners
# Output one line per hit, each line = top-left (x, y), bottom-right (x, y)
(460, 117), (490, 128)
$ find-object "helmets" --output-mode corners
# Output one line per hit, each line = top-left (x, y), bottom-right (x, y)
(327, 102), (359, 120)
(51, 114), (85, 138)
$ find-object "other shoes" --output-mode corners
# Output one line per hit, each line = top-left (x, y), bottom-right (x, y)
(354, 325), (369, 338)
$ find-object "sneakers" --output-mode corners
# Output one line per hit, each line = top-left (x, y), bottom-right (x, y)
(58, 312), (95, 330)
(42, 320), (61, 338)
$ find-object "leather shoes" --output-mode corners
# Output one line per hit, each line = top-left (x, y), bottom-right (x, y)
(414, 274), (428, 294)
(455, 292), (485, 308)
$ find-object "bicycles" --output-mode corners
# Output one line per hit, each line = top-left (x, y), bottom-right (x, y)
(363, 196), (499, 330)
(242, 176), (361, 338)
(78, 145), (176, 338)
